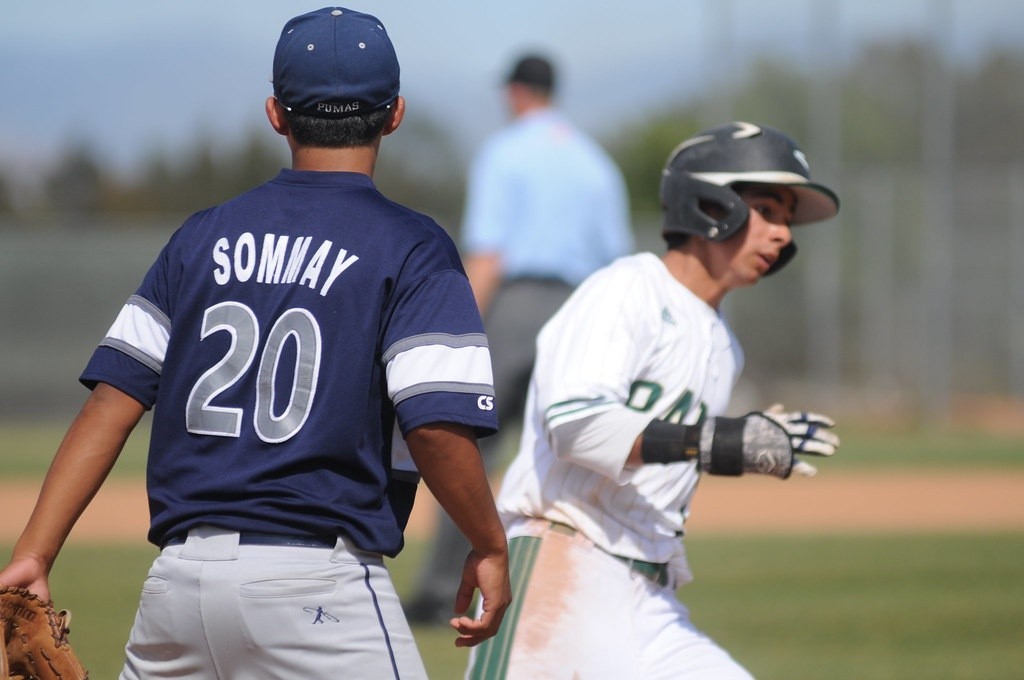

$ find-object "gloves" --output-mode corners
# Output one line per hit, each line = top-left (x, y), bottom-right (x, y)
(697, 404), (841, 481)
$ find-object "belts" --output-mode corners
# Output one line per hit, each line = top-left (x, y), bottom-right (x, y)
(162, 528), (334, 548)
(549, 521), (670, 588)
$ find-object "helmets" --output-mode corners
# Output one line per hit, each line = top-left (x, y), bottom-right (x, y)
(661, 122), (840, 275)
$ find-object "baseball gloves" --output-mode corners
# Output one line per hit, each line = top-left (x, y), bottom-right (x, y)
(0, 587), (89, 680)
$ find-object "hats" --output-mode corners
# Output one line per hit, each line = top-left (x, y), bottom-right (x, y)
(272, 7), (401, 117)
(510, 58), (550, 87)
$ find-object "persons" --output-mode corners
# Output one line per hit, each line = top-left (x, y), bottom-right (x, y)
(402, 55), (638, 626)
(0, 5), (515, 680)
(465, 119), (840, 680)
